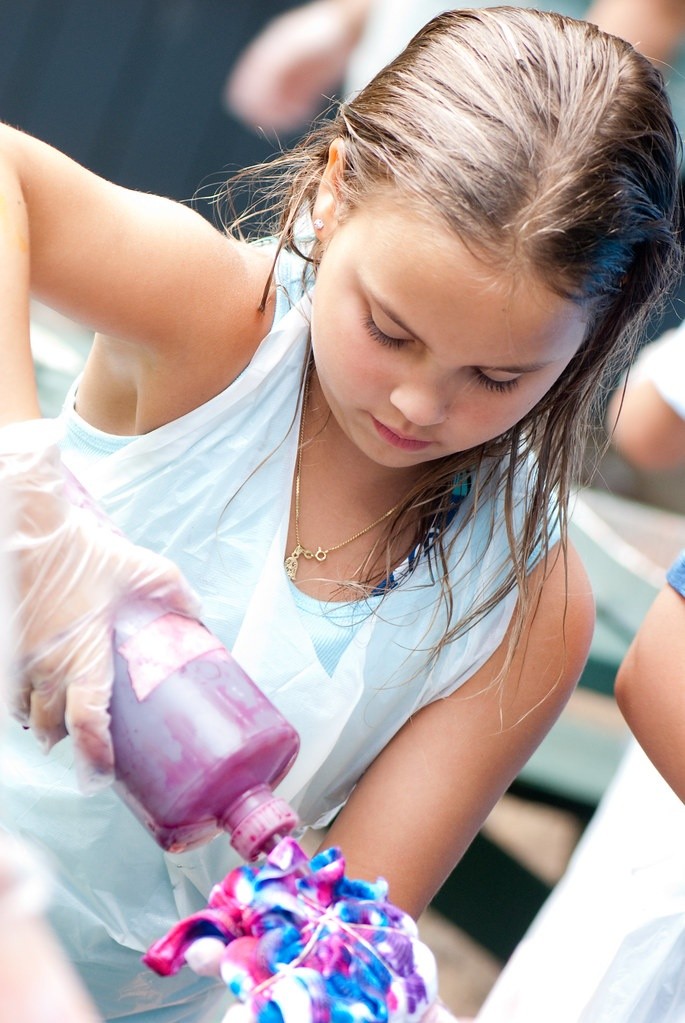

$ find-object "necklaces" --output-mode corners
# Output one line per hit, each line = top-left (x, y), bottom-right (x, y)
(284, 366), (399, 580)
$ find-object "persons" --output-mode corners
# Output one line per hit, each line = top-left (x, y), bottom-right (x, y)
(479, 543), (685, 1023)
(608, 318), (685, 473)
(227, 0), (685, 141)
(0, 5), (679, 1023)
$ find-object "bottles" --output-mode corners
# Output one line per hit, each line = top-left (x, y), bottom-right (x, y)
(55, 461), (301, 863)
